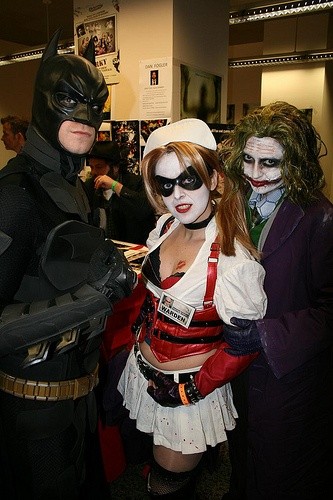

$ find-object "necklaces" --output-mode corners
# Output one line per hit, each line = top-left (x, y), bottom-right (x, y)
(184, 206), (216, 230)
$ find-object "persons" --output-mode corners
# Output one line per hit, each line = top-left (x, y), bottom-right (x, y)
(0, 116), (29, 153)
(116, 118), (267, 500)
(219, 102), (333, 500)
(162, 297), (172, 307)
(0, 26), (137, 500)
(151, 70), (158, 85)
(85, 140), (156, 245)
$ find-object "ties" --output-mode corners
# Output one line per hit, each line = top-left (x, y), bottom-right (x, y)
(249, 201), (264, 231)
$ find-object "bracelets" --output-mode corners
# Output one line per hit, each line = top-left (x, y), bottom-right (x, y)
(179, 384), (189, 404)
(112, 180), (118, 191)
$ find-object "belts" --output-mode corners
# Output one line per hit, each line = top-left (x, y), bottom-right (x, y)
(0, 361), (100, 401)
(134, 341), (201, 389)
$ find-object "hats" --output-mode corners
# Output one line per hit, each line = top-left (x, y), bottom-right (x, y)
(81, 140), (129, 170)
(143, 118), (216, 160)
